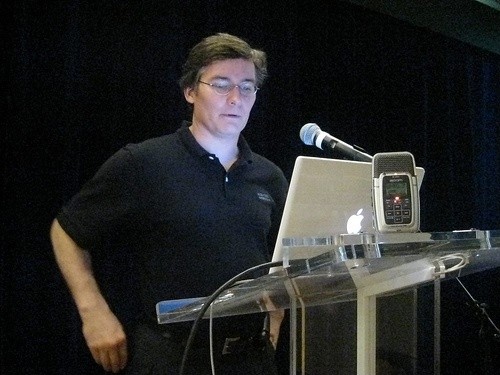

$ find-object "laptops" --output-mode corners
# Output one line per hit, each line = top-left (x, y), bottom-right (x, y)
(269, 156), (425, 276)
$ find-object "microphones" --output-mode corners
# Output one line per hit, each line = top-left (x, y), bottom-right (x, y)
(300, 123), (373, 163)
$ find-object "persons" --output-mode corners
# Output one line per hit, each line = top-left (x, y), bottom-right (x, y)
(49, 32), (290, 375)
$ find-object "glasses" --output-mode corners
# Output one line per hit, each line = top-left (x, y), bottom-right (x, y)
(199, 79), (260, 96)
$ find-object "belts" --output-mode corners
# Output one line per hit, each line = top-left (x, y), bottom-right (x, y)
(144, 314), (263, 355)
